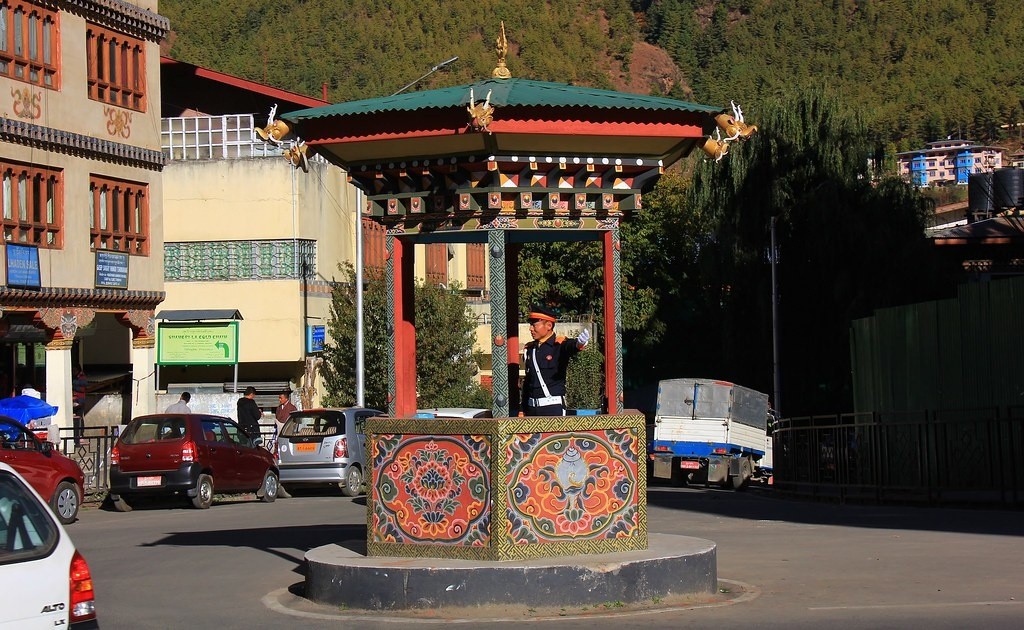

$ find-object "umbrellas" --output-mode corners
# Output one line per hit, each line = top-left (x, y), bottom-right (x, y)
(1, 395), (58, 449)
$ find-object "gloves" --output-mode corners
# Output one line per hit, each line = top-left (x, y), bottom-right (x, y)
(577, 329), (590, 345)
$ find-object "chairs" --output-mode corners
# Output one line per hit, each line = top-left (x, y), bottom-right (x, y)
(163, 429), (181, 440)
(293, 427), (337, 435)
(205, 429), (217, 441)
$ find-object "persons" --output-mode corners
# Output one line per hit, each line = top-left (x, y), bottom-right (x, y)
(164, 391), (191, 414)
(273, 390), (298, 438)
(236, 385), (264, 447)
(517, 302), (589, 417)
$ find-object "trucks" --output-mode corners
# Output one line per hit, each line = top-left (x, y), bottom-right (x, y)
(647, 378), (788, 491)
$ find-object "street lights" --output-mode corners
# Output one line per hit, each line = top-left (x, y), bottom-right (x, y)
(355, 55), (462, 411)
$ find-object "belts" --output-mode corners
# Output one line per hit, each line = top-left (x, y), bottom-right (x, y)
(528, 396), (566, 407)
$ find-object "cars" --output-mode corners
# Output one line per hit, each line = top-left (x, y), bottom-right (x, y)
(0, 414), (87, 526)
(0, 463), (101, 630)
(110, 413), (280, 513)
(415, 406), (492, 421)
(272, 408), (384, 498)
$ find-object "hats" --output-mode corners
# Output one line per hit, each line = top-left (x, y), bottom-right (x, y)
(527, 303), (561, 323)
